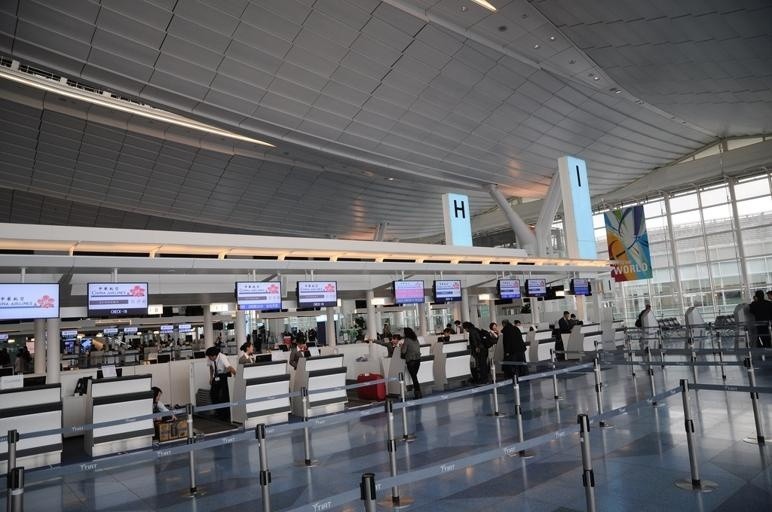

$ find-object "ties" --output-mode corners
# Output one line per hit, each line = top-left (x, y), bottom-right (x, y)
(214, 361), (217, 376)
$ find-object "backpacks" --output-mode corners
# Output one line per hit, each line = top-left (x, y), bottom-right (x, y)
(480, 330), (494, 348)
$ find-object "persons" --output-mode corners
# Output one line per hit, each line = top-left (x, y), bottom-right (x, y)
(454, 320), (464, 334)
(205, 345), (237, 420)
(0, 346), (31, 374)
(383, 323), (390, 337)
(255, 335), (263, 353)
(237, 341), (255, 364)
(638, 304), (652, 344)
(283, 328), (318, 346)
(750, 289), (772, 349)
(486, 322), (500, 367)
(501, 319), (528, 379)
(150, 385), (178, 441)
(447, 323), (455, 334)
(513, 320), (521, 328)
(570, 313), (576, 321)
(462, 321), (489, 387)
(289, 336), (311, 369)
(766, 290), (772, 301)
(558, 311), (576, 361)
(87, 344), (98, 357)
(128, 335), (181, 353)
(370, 333), (402, 357)
(399, 326), (423, 400)
(441, 327), (451, 343)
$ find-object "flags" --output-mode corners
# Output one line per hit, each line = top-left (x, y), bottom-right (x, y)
(603, 203), (654, 283)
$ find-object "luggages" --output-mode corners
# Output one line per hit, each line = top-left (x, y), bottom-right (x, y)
(357, 374), (386, 400)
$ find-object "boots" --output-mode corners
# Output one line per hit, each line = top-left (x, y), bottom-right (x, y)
(413, 391), (422, 399)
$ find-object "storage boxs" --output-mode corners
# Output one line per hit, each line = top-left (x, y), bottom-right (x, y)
(157, 417), (189, 441)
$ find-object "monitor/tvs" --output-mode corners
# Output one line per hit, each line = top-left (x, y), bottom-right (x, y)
(570, 278), (589, 295)
(0, 367), (14, 378)
(23, 375), (46, 388)
(496, 279), (521, 299)
(548, 321), (583, 333)
(256, 354), (272, 364)
(233, 280), (282, 311)
(0, 282), (60, 320)
(87, 282), (148, 316)
(306, 343), (315, 347)
(438, 337), (449, 342)
(295, 281), (337, 308)
(279, 345), (289, 351)
(157, 355), (170, 363)
(525, 279), (547, 296)
(82, 376), (92, 394)
(432, 280), (462, 302)
(391, 280), (425, 304)
(192, 351), (206, 359)
